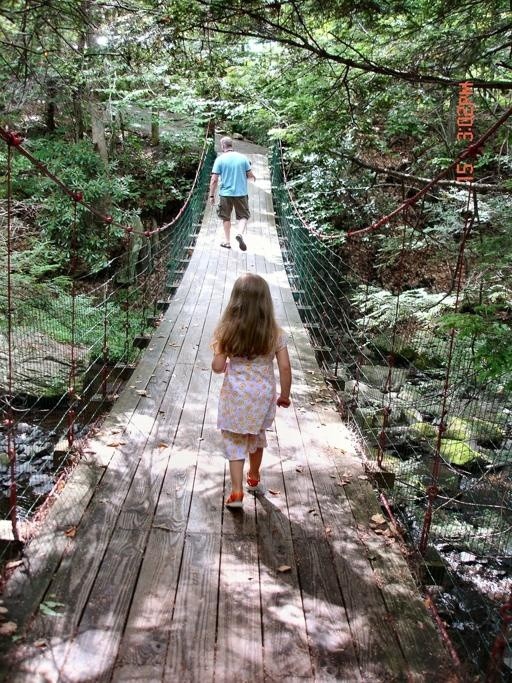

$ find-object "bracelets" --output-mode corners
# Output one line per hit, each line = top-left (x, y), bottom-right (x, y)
(210, 195), (214, 198)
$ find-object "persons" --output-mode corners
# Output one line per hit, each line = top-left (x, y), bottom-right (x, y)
(210, 136), (257, 251)
(209, 271), (291, 509)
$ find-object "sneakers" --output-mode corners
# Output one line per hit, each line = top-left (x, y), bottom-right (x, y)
(245, 470), (261, 491)
(224, 493), (243, 508)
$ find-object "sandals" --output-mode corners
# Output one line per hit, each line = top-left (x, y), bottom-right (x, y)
(235, 234), (246, 250)
(220, 242), (232, 247)
